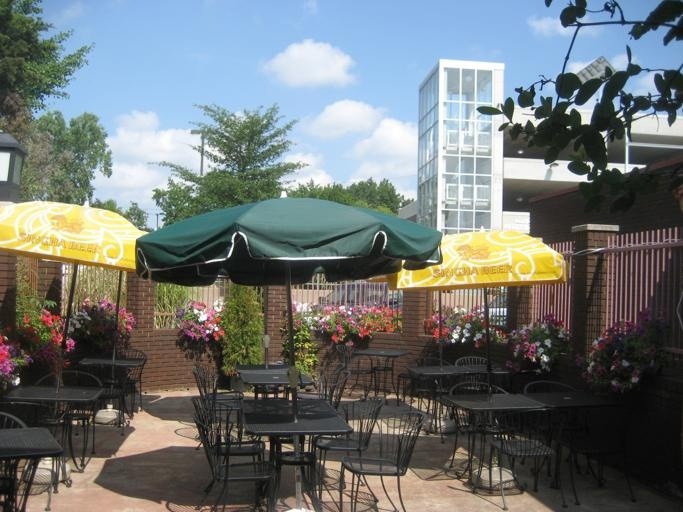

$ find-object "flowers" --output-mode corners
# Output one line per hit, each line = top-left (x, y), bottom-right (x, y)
(176, 291), (405, 376)
(1, 266), (136, 390)
(429, 304), (666, 399)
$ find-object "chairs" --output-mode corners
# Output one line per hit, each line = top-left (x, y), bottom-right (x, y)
(192, 344), (681, 510)
(1, 346), (148, 510)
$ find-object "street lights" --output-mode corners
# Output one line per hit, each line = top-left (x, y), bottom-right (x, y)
(190, 129), (217, 179)
(154, 211), (165, 229)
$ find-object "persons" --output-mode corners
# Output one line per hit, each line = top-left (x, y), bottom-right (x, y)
(135, 196), (440, 420)
(385, 229), (566, 393)
(0, 201), (141, 392)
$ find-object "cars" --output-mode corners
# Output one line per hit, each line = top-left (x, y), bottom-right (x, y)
(308, 281), (404, 333)
(478, 289), (510, 324)
